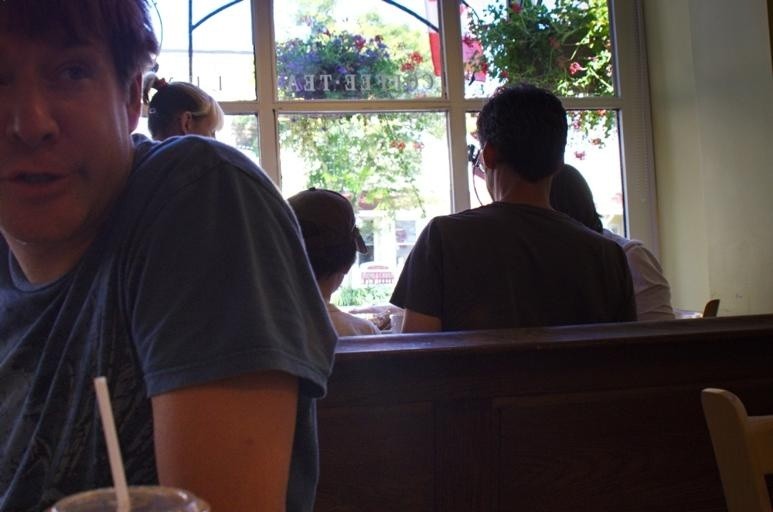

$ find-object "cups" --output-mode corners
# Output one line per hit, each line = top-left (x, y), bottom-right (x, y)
(47, 484), (209, 511)
(389, 313), (403, 334)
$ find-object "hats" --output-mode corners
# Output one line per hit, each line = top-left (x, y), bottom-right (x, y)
(287, 188), (368, 254)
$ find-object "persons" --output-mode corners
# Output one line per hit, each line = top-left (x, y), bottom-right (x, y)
(142, 71), (226, 142)
(287, 186), (382, 337)
(549, 162), (676, 321)
(0, 0), (344, 512)
(387, 82), (639, 334)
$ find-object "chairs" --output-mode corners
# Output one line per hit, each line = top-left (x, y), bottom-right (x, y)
(701, 389), (773, 512)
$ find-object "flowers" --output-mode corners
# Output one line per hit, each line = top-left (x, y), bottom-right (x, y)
(276, 14), (433, 162)
(462, 0), (617, 159)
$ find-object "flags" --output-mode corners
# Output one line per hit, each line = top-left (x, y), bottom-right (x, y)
(426, 1), (489, 80)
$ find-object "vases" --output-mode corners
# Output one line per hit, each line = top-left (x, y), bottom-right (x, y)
(300, 77), (365, 101)
